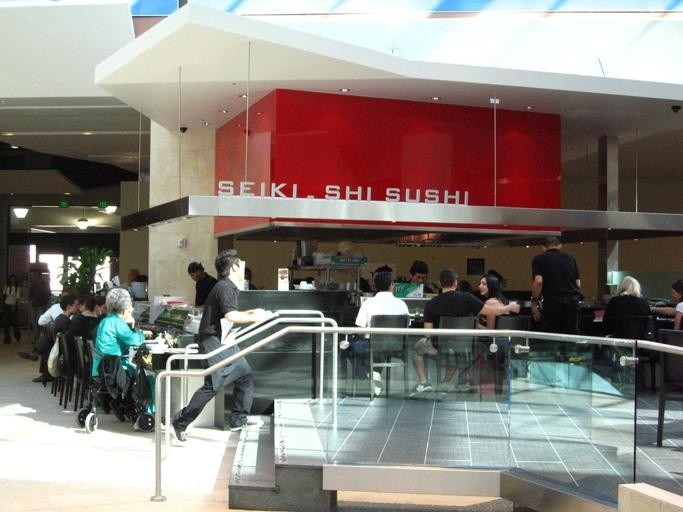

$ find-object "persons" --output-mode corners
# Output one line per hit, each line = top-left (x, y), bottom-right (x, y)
(170, 248), (268, 447)
(188, 261), (218, 307)
(245, 268), (265, 290)
(0, 274), (21, 345)
(17, 268), (164, 431)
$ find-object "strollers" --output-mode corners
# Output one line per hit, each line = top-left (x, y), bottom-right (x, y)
(78, 354), (154, 433)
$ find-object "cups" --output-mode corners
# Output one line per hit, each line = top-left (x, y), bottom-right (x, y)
(311, 279), (357, 291)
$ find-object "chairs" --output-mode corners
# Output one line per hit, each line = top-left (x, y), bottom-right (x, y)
(611, 307), (683, 447)
(355, 314), (411, 402)
(424, 315), (478, 402)
(37, 330), (154, 412)
(477, 315), (533, 395)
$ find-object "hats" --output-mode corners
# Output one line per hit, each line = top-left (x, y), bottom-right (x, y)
(105, 287), (131, 311)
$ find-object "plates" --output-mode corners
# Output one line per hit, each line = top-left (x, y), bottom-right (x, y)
(130, 281), (146, 300)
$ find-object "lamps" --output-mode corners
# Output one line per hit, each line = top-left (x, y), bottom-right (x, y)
(105, 206), (117, 213)
(13, 208), (28, 219)
(76, 208), (89, 230)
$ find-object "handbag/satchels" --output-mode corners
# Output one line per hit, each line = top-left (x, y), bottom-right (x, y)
(46, 331), (63, 378)
(571, 301), (597, 323)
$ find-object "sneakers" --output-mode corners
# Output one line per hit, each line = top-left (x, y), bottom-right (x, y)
(434, 382), (450, 398)
(170, 422), (188, 444)
(402, 381), (433, 399)
(370, 369), (383, 397)
(228, 418), (264, 432)
